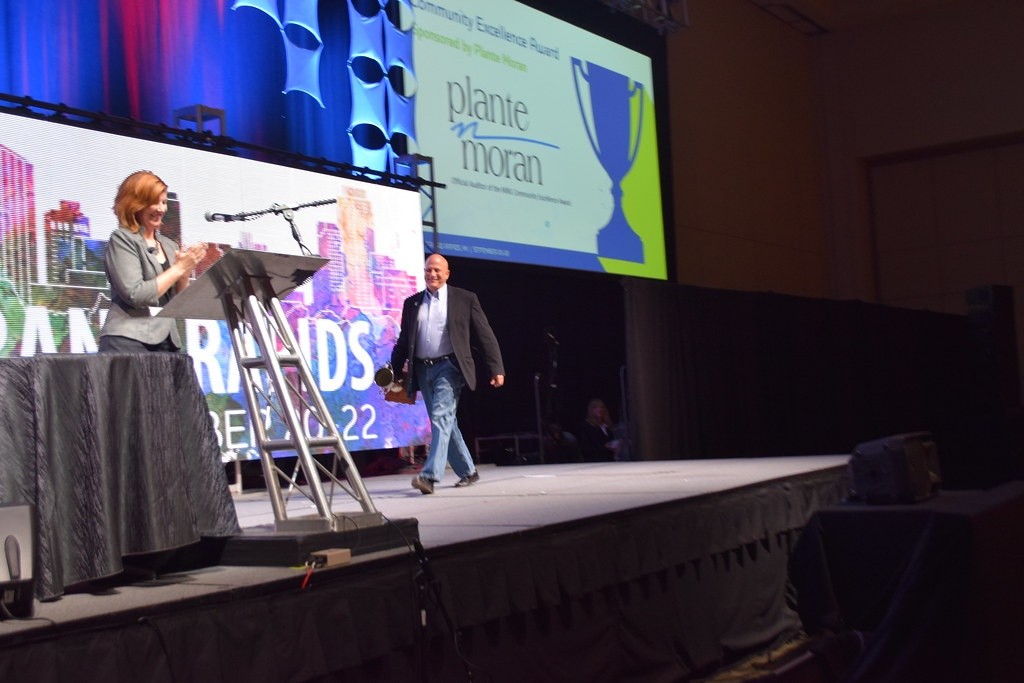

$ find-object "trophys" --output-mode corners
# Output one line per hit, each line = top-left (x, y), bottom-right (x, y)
(374, 363), (416, 405)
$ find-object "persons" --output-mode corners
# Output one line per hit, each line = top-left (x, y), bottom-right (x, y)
(96, 171), (208, 355)
(390, 254), (504, 494)
(576, 398), (623, 463)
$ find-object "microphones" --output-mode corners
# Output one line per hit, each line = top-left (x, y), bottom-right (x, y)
(148, 247), (155, 253)
(206, 212), (247, 223)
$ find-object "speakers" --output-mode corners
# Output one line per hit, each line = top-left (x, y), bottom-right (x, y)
(0, 504), (35, 621)
(847, 431), (942, 507)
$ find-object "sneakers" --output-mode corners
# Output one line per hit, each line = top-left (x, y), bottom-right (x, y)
(412, 476), (434, 494)
(455, 470), (479, 487)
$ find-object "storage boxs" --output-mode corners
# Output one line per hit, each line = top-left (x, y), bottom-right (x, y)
(474, 435), (540, 466)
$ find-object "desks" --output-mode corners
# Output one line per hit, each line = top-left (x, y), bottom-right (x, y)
(0, 351), (242, 600)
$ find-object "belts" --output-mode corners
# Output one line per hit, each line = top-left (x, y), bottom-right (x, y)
(414, 352), (455, 367)
